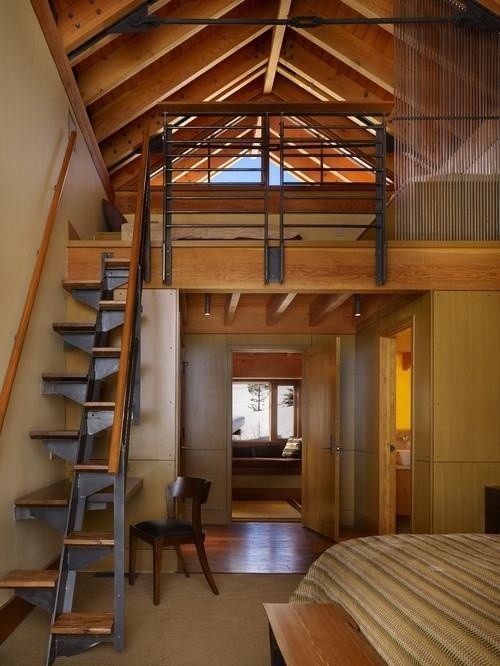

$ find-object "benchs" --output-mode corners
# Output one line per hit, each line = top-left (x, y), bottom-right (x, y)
(262, 603), (388, 666)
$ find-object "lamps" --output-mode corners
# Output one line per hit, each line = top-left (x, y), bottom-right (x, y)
(203, 293), (211, 316)
(354, 294), (361, 317)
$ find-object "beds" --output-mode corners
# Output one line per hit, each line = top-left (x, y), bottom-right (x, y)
(233, 457), (302, 500)
(289, 533), (500, 666)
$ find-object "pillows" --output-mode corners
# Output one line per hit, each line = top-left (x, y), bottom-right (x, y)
(282, 436), (302, 458)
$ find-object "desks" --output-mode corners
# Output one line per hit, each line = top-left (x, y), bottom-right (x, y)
(14, 477), (144, 530)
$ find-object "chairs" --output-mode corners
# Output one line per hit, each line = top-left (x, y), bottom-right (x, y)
(129, 477), (220, 606)
